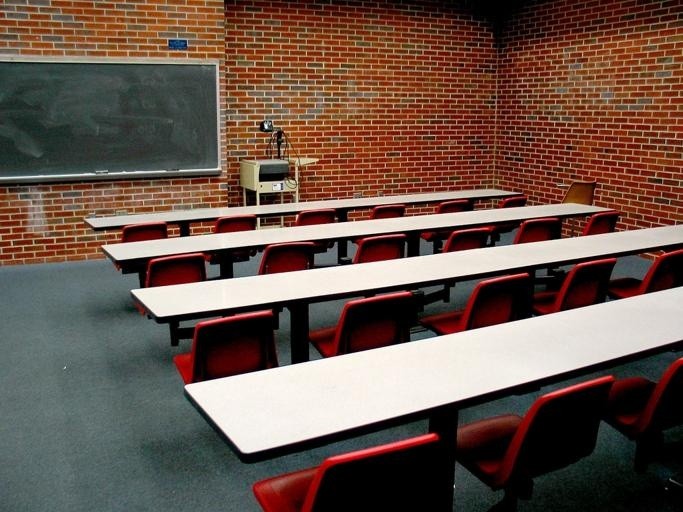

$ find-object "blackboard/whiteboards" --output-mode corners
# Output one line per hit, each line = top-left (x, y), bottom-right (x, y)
(0, 54), (222, 184)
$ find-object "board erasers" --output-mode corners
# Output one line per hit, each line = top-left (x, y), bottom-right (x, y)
(95, 170), (108, 174)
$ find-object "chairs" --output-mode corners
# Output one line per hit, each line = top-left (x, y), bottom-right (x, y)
(529, 257), (618, 315)
(255, 242), (315, 275)
(442, 225), (496, 255)
(457, 376), (615, 512)
(340, 233), (406, 265)
(351, 204), (407, 245)
(128, 252), (207, 349)
(309, 290), (414, 360)
(513, 218), (561, 245)
(418, 272), (531, 337)
(204, 215), (258, 278)
(581, 211), (628, 236)
(171, 310), (280, 383)
(607, 249), (682, 299)
(562, 182), (597, 205)
(108, 221), (168, 289)
(292, 209), (336, 266)
(420, 199), (470, 256)
(496, 196), (528, 244)
(253, 433), (439, 512)
(601, 359), (683, 474)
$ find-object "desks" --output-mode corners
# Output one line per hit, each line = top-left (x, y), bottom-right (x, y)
(84, 189), (526, 236)
(101, 201), (618, 278)
(184, 286), (683, 512)
(129, 224), (682, 365)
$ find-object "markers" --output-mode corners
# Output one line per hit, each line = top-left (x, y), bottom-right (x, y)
(167, 168), (179, 171)
